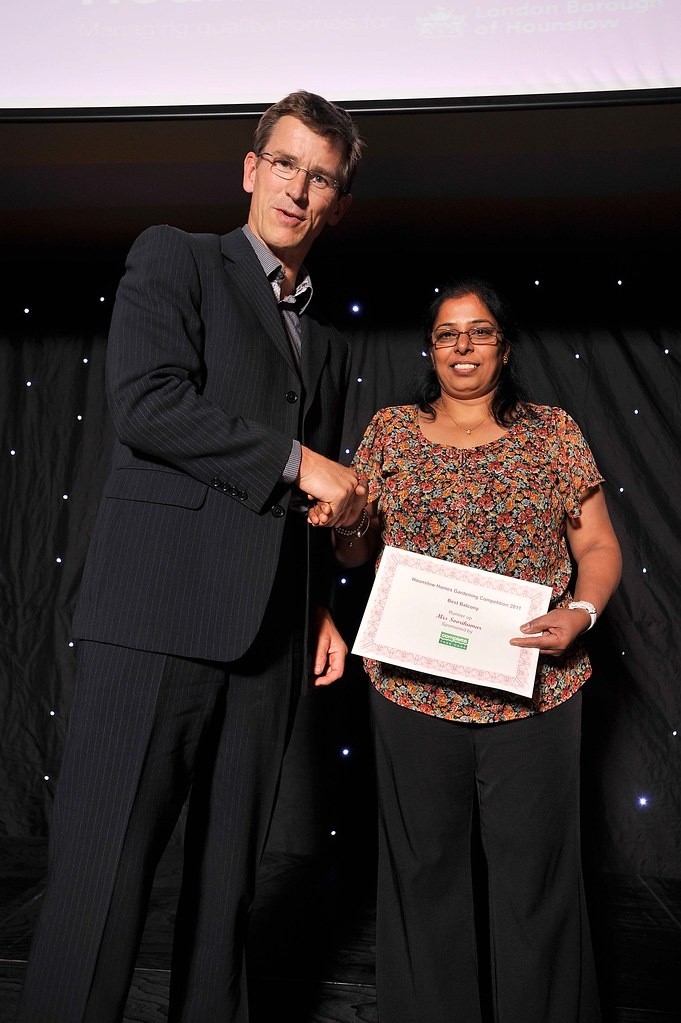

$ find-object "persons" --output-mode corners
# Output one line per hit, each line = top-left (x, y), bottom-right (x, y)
(306, 277), (625, 1022)
(16, 90), (364, 1023)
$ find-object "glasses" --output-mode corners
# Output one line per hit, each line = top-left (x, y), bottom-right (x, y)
(430, 326), (504, 350)
(257, 150), (344, 198)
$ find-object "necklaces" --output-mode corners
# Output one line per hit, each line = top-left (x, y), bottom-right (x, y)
(440, 396), (492, 435)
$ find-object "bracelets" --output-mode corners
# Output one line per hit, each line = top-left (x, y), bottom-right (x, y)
(335, 507), (371, 548)
(568, 600), (597, 636)
